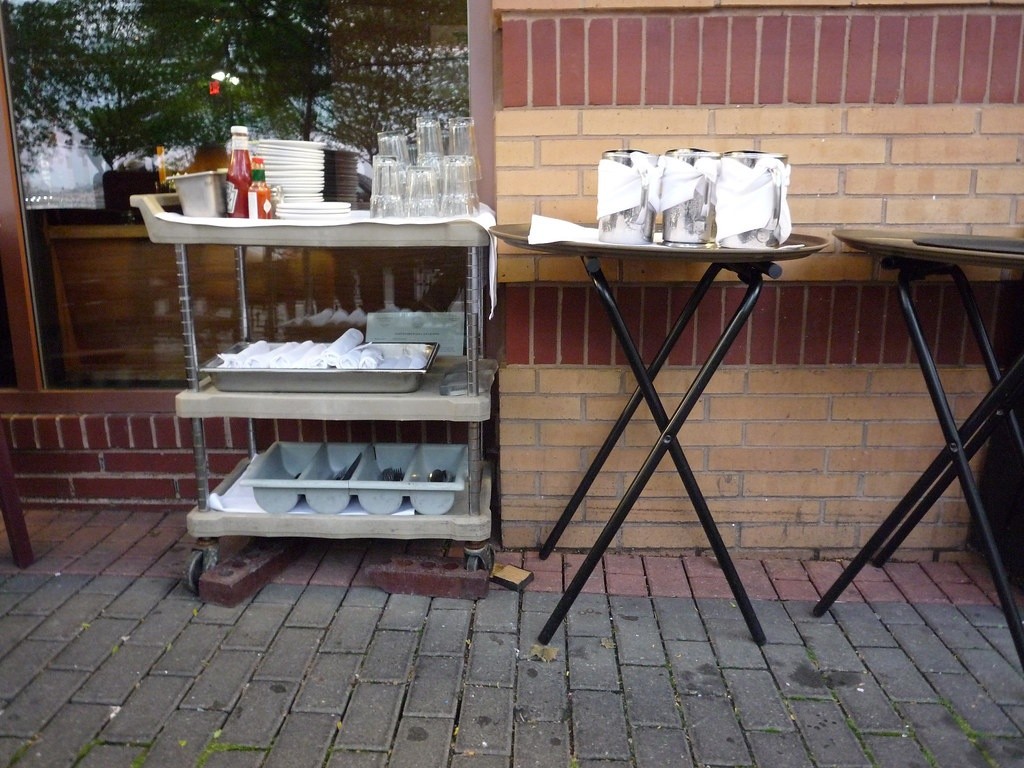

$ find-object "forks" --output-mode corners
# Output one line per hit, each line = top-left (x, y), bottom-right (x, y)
(378, 465), (403, 482)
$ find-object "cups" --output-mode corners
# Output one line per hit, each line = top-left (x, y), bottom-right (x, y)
(372, 115), (482, 221)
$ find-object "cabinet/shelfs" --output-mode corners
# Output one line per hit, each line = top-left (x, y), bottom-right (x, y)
(129, 193), (496, 596)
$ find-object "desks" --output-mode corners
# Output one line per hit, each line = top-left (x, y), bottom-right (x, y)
(489, 222), (831, 646)
(812, 227), (1024, 672)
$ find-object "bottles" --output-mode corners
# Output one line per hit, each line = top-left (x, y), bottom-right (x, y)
(226, 125), (252, 217)
(248, 157), (272, 220)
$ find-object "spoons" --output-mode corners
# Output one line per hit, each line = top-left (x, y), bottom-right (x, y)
(427, 467), (456, 485)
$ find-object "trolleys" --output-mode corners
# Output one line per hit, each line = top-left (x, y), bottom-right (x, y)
(129, 195), (498, 596)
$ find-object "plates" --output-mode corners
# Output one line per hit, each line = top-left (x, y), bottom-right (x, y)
(250, 137), (360, 220)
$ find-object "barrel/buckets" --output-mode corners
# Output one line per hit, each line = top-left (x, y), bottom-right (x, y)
(724, 149), (786, 247)
(661, 147), (718, 248)
(598, 150), (656, 242)
